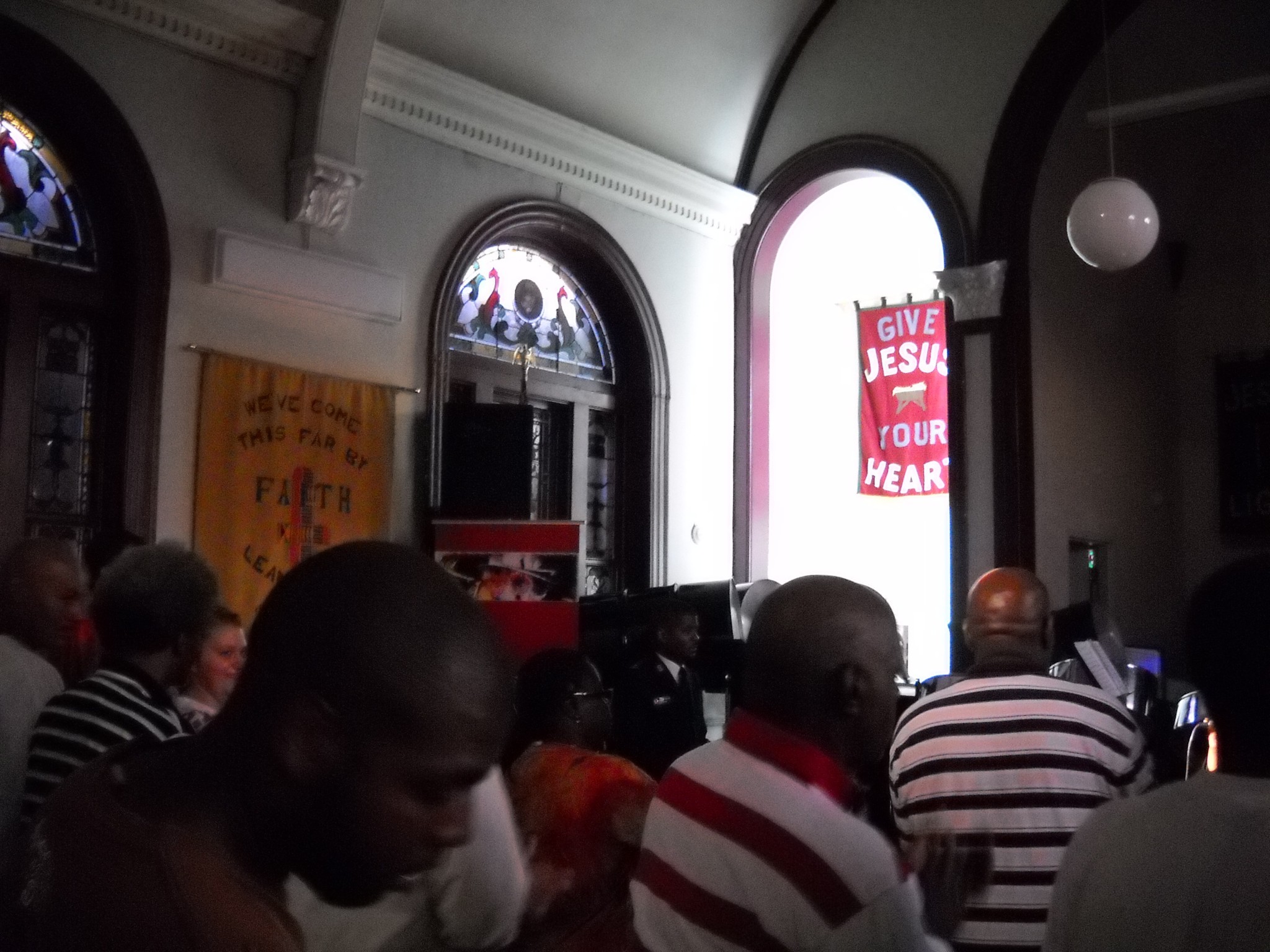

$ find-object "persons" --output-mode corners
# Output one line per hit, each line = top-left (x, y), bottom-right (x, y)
(172, 608), (249, 734)
(15, 541), (224, 827)
(284, 761), (533, 951)
(0, 541), (515, 951)
(503, 651), (656, 951)
(626, 575), (990, 951)
(1037, 552), (1270, 952)
(889, 567), (1153, 952)
(0, 536), (89, 838)
(611, 596), (708, 787)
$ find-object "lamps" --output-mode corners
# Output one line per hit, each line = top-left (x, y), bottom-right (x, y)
(1064, 0), (1160, 270)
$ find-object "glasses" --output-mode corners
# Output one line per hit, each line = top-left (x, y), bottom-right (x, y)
(559, 686), (616, 710)
